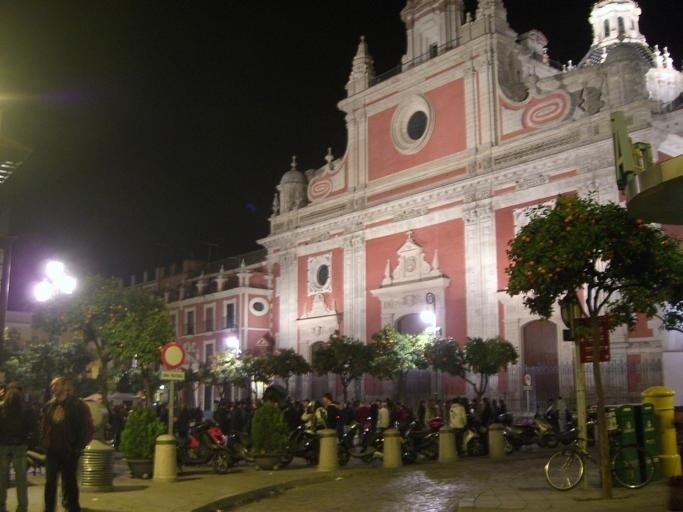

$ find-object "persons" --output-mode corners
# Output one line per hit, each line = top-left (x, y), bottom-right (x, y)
(281, 394), (412, 443)
(547, 396), (566, 432)
(156, 403), (168, 425)
(109, 402), (132, 448)
(0, 375), (94, 512)
(177, 400), (262, 453)
(415, 396), (507, 453)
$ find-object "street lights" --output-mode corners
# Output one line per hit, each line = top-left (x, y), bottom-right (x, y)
(31, 259), (79, 344)
(224, 335), (242, 402)
(419, 309), (438, 394)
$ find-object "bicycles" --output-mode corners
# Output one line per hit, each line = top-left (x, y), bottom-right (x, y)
(544, 417), (656, 491)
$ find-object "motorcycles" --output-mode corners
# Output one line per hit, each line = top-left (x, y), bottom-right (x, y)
(174, 396), (599, 474)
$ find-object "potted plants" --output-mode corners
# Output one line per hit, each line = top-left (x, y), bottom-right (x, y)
(119, 402), (167, 478)
(246, 403), (288, 468)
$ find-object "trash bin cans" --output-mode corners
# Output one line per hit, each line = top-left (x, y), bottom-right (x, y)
(604, 402), (663, 486)
